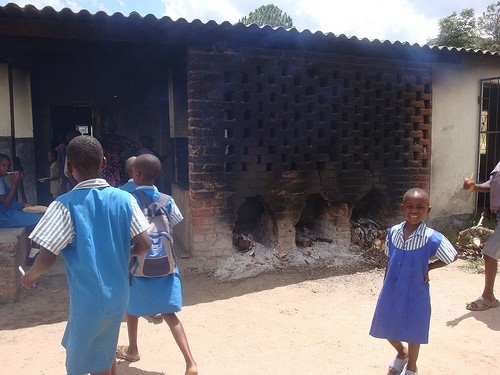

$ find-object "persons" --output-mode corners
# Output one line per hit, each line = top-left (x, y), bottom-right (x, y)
(19, 136), (152, 375)
(368, 187), (458, 375)
(36, 150), (64, 198)
(115, 155), (198, 375)
(463, 159), (500, 312)
(0, 153), (48, 265)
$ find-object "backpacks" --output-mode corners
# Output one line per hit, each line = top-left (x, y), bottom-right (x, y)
(129, 190), (175, 278)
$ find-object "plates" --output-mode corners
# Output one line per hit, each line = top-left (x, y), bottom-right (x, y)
(38, 178), (48, 182)
(23, 206), (49, 213)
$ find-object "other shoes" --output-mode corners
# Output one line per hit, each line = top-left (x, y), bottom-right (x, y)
(26, 252), (40, 266)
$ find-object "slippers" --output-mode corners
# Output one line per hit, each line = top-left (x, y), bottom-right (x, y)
(387, 355), (408, 375)
(404, 368), (418, 375)
(115, 345), (140, 362)
(466, 297), (499, 311)
